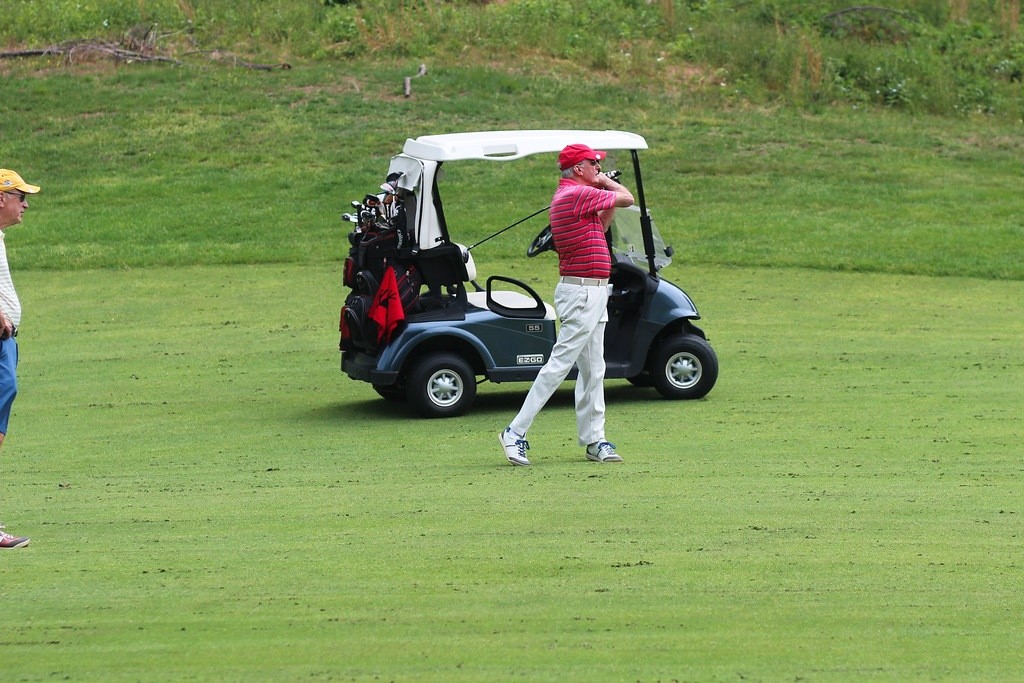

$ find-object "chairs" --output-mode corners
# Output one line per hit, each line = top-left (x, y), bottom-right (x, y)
(455, 243), (557, 320)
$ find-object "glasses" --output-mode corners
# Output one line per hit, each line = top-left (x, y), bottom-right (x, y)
(5, 191), (26, 203)
(577, 160), (600, 166)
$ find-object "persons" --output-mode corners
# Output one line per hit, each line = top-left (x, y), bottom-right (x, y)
(498, 144), (634, 466)
(0, 169), (40, 549)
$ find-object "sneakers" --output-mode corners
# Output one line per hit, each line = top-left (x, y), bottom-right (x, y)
(497, 426), (532, 467)
(585, 440), (623, 463)
(0, 525), (31, 549)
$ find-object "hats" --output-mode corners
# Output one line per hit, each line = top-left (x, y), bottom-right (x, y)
(556, 143), (607, 172)
(0, 168), (41, 194)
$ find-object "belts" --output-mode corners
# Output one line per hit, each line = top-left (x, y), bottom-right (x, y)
(559, 275), (610, 287)
(1, 329), (19, 341)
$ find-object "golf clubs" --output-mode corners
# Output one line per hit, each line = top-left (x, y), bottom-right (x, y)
(461, 170), (623, 264)
(340, 172), (405, 234)
